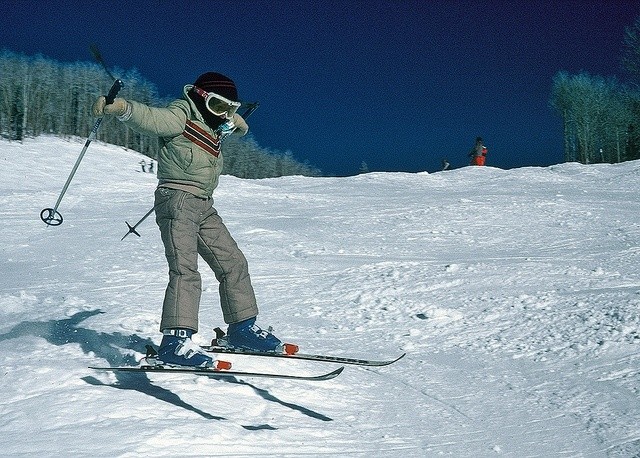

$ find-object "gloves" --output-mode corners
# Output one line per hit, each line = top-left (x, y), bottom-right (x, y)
(92, 96), (129, 118)
(233, 114), (249, 137)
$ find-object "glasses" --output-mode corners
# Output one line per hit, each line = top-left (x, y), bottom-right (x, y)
(205, 93), (241, 120)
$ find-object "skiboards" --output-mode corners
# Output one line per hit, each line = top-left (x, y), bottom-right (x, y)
(87, 345), (406, 380)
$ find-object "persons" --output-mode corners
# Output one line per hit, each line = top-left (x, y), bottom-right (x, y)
(139, 159), (146, 172)
(92, 70), (283, 370)
(148, 160), (154, 172)
(468, 136), (486, 165)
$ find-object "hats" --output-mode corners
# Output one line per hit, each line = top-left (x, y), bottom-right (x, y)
(192, 72), (237, 129)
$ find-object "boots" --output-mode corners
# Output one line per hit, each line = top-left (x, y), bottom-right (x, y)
(159, 327), (212, 366)
(226, 317), (283, 352)
